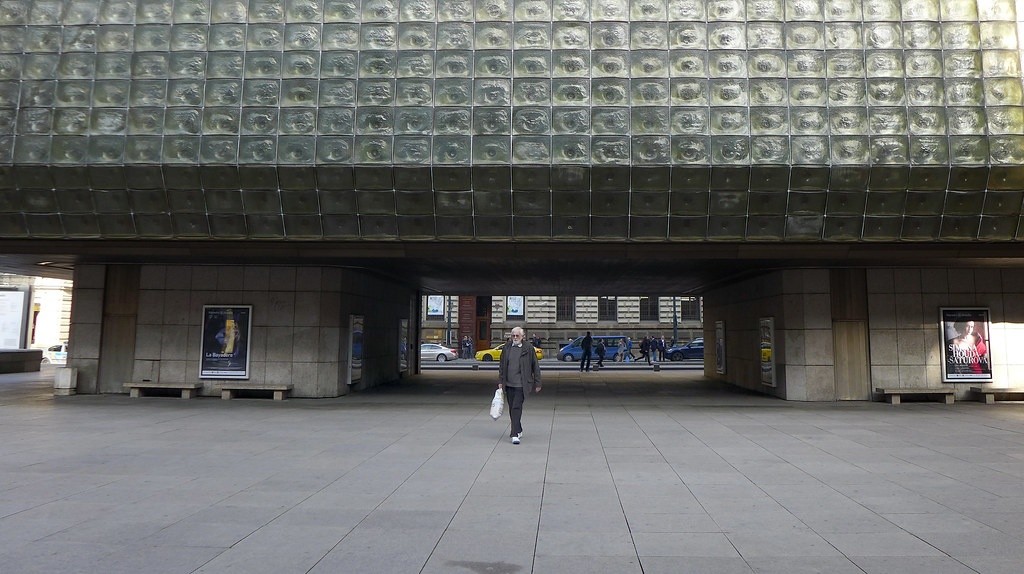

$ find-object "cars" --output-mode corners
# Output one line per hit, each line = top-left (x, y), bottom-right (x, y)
(474, 342), (544, 361)
(665, 338), (704, 362)
(421, 343), (459, 363)
(35, 340), (68, 364)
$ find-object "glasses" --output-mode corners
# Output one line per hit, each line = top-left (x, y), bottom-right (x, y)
(513, 335), (519, 337)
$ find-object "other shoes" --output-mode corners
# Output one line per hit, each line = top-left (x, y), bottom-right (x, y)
(512, 437), (520, 444)
(518, 433), (522, 438)
(580, 369), (590, 372)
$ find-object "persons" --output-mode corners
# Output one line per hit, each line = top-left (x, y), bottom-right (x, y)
(617, 339), (625, 364)
(634, 335), (667, 365)
(580, 331), (593, 372)
(568, 337), (573, 344)
(498, 327), (542, 443)
(596, 339), (605, 367)
(628, 337), (635, 359)
(669, 336), (675, 347)
(462, 336), (473, 359)
(946, 317), (988, 374)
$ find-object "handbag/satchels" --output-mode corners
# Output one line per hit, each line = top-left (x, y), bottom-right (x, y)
(490, 388), (505, 420)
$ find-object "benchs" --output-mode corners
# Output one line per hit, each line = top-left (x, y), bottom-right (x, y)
(970, 386), (1024, 405)
(214, 383), (294, 401)
(122, 381), (204, 399)
(875, 387), (959, 405)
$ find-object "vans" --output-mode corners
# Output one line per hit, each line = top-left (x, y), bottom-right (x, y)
(556, 336), (629, 361)
